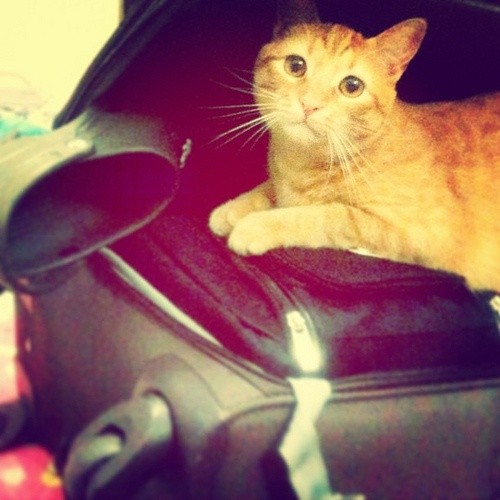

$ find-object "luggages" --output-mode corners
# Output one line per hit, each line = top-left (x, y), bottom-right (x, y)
(0, 1), (500, 500)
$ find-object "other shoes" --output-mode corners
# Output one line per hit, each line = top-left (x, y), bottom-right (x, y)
(0, 107), (182, 280)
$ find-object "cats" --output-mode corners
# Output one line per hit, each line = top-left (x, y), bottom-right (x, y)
(207, 1), (500, 313)
